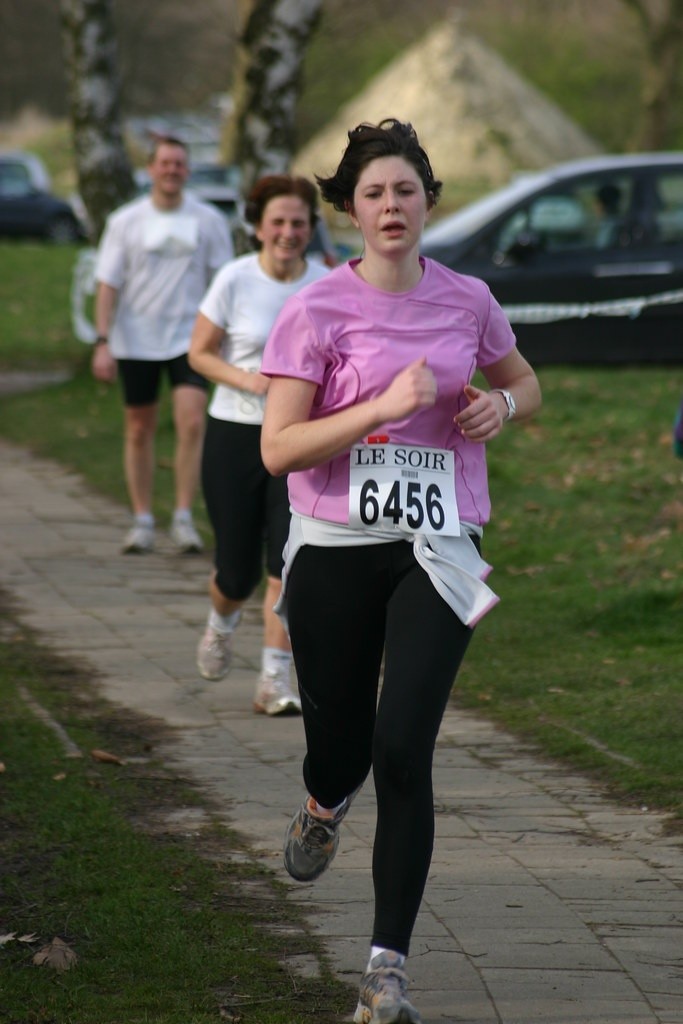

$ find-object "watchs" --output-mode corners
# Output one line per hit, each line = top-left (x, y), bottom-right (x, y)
(489, 389), (516, 423)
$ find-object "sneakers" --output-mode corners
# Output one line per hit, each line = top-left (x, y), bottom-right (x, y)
(196, 612), (243, 681)
(283, 784), (363, 881)
(353, 950), (421, 1024)
(254, 669), (302, 715)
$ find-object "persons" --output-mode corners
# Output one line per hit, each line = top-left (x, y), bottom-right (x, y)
(592, 184), (623, 249)
(189, 177), (331, 718)
(260, 120), (541, 1024)
(93, 138), (235, 554)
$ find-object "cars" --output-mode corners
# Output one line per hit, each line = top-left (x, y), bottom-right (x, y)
(0, 154), (87, 248)
(419, 152), (683, 367)
(135, 112), (246, 220)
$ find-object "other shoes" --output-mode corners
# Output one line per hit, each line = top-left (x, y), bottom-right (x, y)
(161, 519), (202, 553)
(121, 521), (153, 553)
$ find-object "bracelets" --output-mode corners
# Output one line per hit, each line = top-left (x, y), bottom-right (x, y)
(96, 337), (107, 345)
